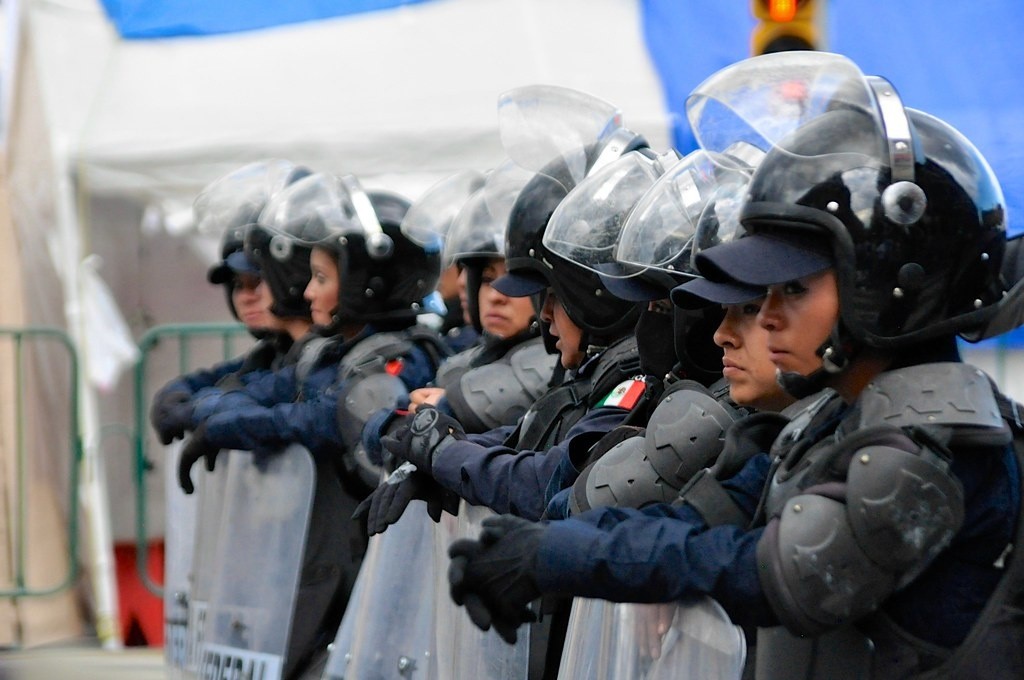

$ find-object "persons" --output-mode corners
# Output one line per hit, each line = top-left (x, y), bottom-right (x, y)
(150, 106), (1024, 680)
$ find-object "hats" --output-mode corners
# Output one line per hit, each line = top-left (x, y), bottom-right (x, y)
(695, 227), (838, 292)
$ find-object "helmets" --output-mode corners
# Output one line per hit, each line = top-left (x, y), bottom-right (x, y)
(734, 71), (1008, 376)
(205, 139), (758, 329)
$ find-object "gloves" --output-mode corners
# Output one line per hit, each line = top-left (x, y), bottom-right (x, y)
(148, 369), (246, 494)
(447, 514), (552, 645)
(349, 401), (466, 536)
